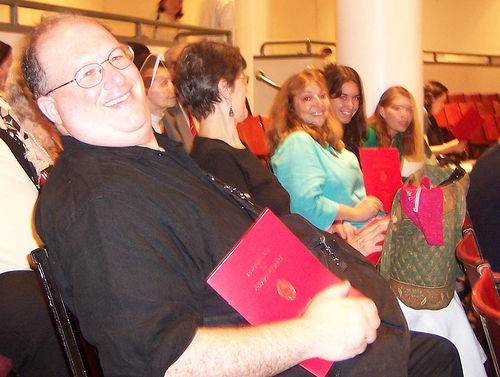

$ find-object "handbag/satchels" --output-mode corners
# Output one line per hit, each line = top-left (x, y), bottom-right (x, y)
(376, 163), (470, 310)
(248, 205), (410, 377)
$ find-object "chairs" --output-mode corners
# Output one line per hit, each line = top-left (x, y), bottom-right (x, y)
(434, 91), (499, 160)
(31, 245), (105, 377)
(455, 213), (500, 377)
(236, 114), (278, 170)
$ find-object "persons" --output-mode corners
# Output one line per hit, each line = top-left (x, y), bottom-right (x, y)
(170, 40), (489, 376)
(316, 47), (335, 72)
(0, 93), (101, 377)
(304, 64), (411, 190)
(0, 40), (18, 100)
(21, 15), (465, 376)
(420, 79), (468, 172)
(467, 131), (500, 321)
(122, 31), (199, 167)
(265, 68), (396, 234)
(351, 85), (433, 188)
(149, 1), (188, 66)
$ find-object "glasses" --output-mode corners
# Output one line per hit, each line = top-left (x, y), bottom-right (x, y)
(44, 44), (135, 97)
